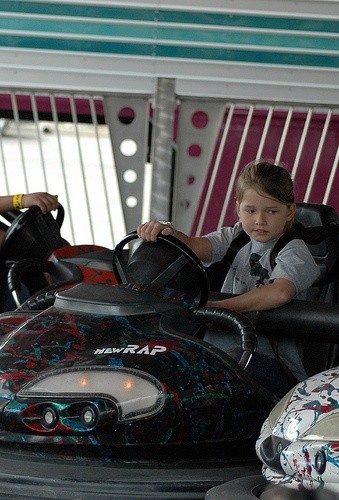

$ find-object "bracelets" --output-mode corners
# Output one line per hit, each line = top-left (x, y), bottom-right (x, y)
(13, 194), (23, 208)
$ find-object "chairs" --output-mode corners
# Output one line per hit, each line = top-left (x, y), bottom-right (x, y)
(288, 203), (338, 376)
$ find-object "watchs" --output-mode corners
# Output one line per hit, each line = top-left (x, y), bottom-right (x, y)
(158, 220), (176, 236)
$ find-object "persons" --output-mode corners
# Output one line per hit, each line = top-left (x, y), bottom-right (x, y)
(134, 161), (319, 406)
(0, 192), (59, 214)
